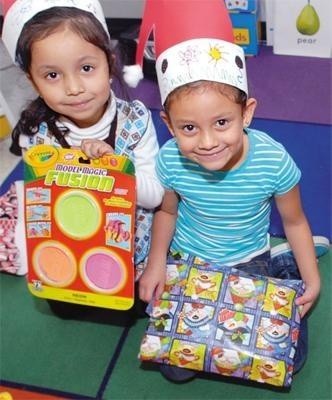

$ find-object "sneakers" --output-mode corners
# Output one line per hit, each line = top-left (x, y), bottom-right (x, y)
(270, 236), (329, 263)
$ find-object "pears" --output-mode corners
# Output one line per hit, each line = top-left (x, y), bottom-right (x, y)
(296, 0), (320, 35)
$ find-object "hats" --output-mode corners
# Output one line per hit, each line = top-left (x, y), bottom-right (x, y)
(1, 1), (111, 70)
(122, 0), (249, 111)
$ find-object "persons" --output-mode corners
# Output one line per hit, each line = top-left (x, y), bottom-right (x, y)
(1, 0), (165, 285)
(138, 37), (329, 383)
